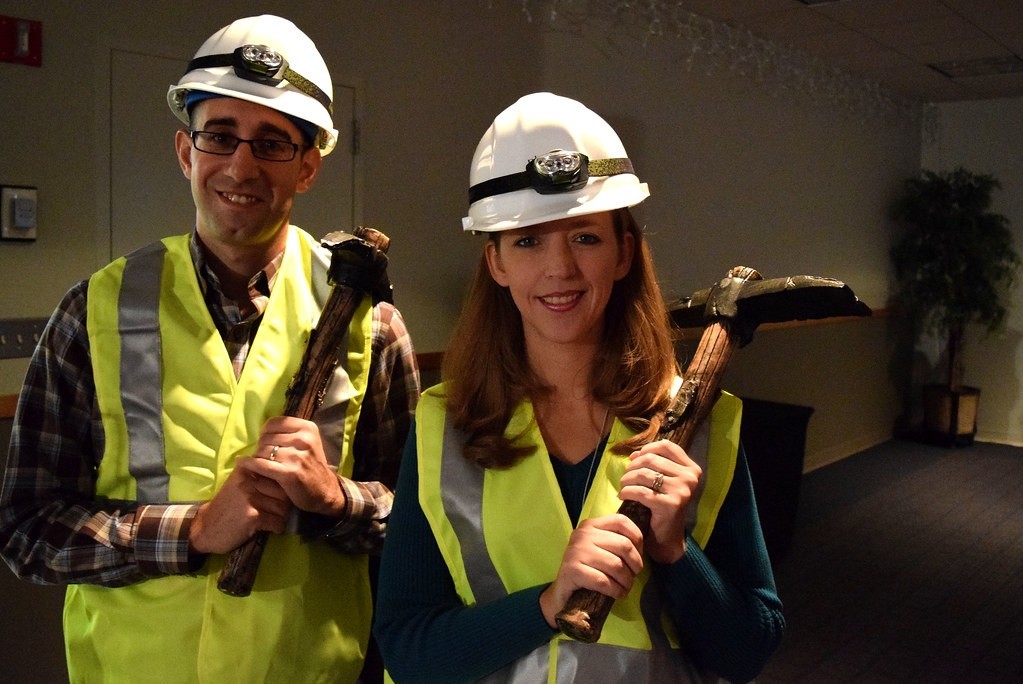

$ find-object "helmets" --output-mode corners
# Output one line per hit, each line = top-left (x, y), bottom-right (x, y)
(167, 14), (339, 158)
(461, 91), (651, 236)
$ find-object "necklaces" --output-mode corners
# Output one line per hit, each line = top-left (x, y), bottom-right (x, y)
(566, 365), (631, 534)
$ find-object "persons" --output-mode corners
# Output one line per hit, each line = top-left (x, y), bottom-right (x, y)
(372, 90), (794, 684)
(0, 11), (439, 684)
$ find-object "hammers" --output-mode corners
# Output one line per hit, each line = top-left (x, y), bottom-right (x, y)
(216, 224), (397, 602)
(549, 265), (882, 647)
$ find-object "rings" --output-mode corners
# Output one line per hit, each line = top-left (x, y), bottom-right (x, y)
(650, 470), (667, 495)
(268, 443), (281, 461)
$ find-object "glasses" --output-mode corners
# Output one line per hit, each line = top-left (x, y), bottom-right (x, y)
(188, 130), (309, 161)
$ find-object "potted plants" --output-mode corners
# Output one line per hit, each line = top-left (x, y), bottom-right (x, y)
(881, 164), (1020, 448)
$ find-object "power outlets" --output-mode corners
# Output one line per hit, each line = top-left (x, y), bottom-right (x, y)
(0, 186), (38, 240)
